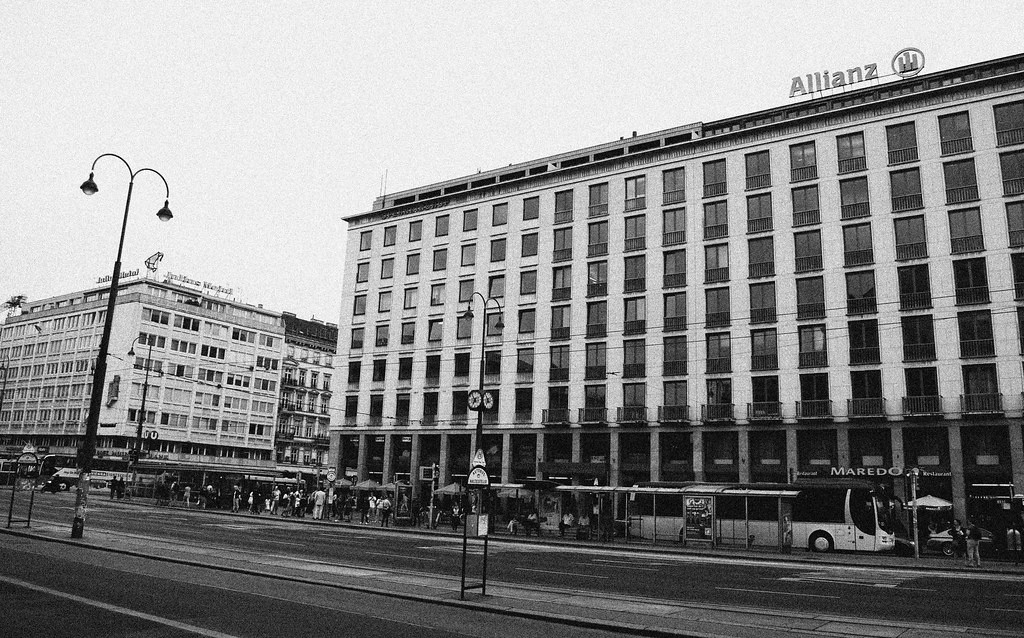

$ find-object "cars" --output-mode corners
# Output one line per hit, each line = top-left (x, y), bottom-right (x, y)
(926, 526), (998, 557)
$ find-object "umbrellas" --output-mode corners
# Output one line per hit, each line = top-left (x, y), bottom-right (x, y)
(334, 478), (352, 489)
(377, 483), (406, 494)
(433, 482), (467, 500)
(904, 494), (953, 521)
(496, 488), (534, 513)
(350, 479), (380, 491)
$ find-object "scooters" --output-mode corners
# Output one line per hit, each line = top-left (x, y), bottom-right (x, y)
(41, 480), (59, 494)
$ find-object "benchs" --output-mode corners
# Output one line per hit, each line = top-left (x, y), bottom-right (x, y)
(716, 536), (756, 549)
(563, 527), (592, 540)
(658, 534), (683, 543)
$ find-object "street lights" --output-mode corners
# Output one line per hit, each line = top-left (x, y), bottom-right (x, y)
(69, 154), (173, 545)
(464, 292), (505, 537)
(127, 336), (153, 498)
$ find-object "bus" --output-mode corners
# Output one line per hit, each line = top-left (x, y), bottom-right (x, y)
(37, 454), (165, 492)
(628, 482), (904, 553)
(243, 476), (307, 496)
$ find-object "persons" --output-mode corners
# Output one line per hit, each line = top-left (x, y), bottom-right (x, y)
(412, 492), (424, 527)
(576, 513), (592, 539)
(168, 481), (221, 509)
(310, 486), (392, 528)
(451, 500), (462, 531)
(110, 475), (125, 499)
(527, 509), (537, 524)
(949, 518), (981, 567)
(558, 510), (574, 536)
(54, 474), (61, 491)
(230, 485), (308, 518)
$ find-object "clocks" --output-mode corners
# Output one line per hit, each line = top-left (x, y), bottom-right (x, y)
(467, 389), (483, 411)
(481, 390), (495, 412)
